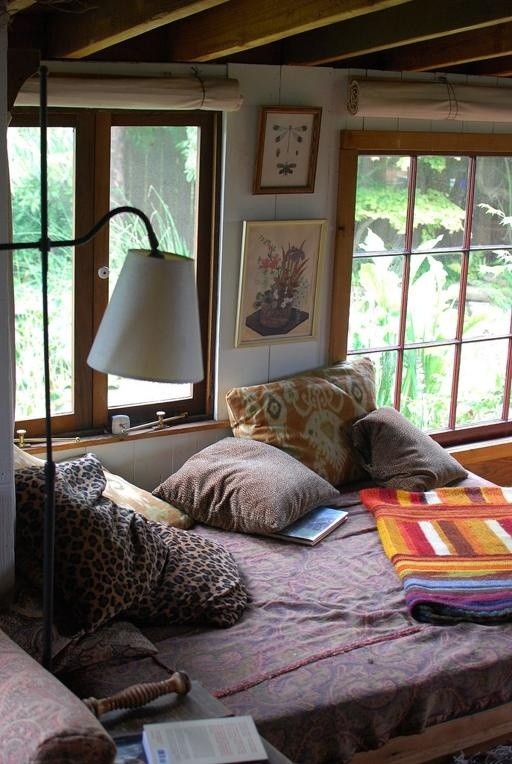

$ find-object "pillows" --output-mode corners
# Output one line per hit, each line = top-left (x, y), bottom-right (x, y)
(151, 436), (341, 534)
(226, 356), (377, 486)
(78, 452), (198, 529)
(351, 406), (469, 492)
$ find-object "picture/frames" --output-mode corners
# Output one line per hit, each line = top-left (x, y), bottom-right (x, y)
(235, 218), (328, 349)
(252, 105), (323, 193)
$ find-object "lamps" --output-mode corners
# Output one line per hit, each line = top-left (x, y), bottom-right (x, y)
(1, 66), (204, 670)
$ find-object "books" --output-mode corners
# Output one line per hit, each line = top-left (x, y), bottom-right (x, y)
(276, 506), (350, 541)
(141, 712), (270, 763)
(264, 517), (348, 546)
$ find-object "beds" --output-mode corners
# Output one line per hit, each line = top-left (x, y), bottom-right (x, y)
(0, 463), (511, 764)
